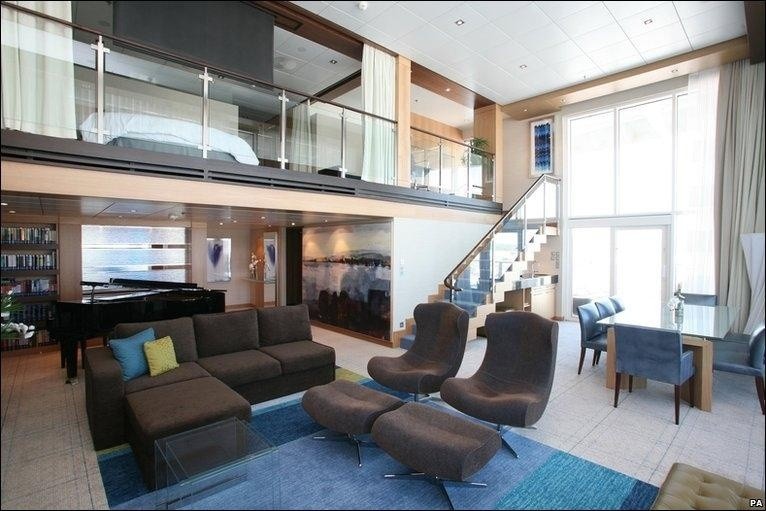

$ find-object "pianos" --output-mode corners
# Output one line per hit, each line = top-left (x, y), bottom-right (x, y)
(54, 278), (227, 386)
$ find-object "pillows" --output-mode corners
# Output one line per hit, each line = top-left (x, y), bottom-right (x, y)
(109, 327), (179, 383)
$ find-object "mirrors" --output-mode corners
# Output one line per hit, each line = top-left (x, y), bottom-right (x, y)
(263, 232), (277, 308)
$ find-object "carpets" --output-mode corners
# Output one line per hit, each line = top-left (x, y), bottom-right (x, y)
(96, 376), (661, 510)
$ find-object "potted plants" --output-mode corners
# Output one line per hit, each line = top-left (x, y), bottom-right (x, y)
(466, 136), (490, 164)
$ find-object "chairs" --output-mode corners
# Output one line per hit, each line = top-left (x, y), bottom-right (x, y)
(366, 301), (559, 458)
(577, 291), (765, 425)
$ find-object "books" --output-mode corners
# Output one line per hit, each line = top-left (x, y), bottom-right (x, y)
(0, 226), (55, 352)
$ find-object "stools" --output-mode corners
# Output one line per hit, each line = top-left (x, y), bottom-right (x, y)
(650, 461), (766, 511)
(302, 378), (503, 511)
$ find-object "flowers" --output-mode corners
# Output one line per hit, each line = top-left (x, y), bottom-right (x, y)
(248, 250), (263, 275)
(0, 289), (35, 340)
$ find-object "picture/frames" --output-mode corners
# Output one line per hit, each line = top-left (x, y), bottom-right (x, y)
(208, 237), (231, 284)
(527, 114), (555, 179)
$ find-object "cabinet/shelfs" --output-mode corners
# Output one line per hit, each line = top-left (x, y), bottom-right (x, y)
(1, 220), (59, 357)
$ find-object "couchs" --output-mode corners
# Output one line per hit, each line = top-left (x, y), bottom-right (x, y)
(86, 304), (337, 492)
